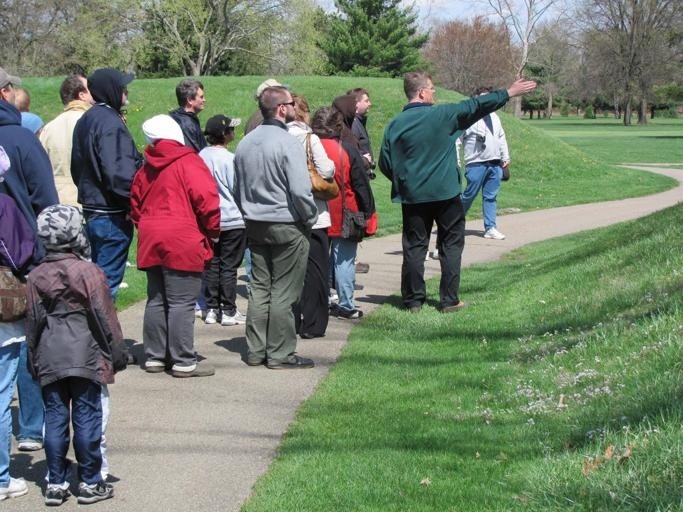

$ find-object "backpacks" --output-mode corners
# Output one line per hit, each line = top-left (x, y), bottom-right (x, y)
(339, 139), (366, 242)
(306, 131), (338, 200)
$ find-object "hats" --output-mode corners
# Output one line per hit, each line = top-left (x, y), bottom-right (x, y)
(0, 67), (22, 90)
(37, 204), (94, 262)
(87, 68), (135, 88)
(256, 78), (281, 97)
(204, 115), (240, 138)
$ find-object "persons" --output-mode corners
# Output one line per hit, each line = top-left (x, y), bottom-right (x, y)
(36, 74), (96, 214)
(379, 71), (538, 313)
(26, 206), (129, 506)
(165, 80), (210, 319)
(131, 115), (222, 377)
(14, 87), (45, 148)
(287, 94), (337, 339)
(307, 107), (377, 320)
(0, 147), (36, 501)
(244, 80), (285, 137)
(71, 69), (144, 365)
(0, 69), (59, 449)
(329, 96), (357, 132)
(344, 90), (376, 273)
(234, 86), (319, 369)
(195, 114), (250, 327)
(452, 88), (510, 240)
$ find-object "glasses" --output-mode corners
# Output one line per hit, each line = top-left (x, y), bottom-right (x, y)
(278, 100), (296, 108)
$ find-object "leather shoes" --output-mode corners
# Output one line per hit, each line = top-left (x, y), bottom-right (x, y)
(407, 303), (422, 312)
(353, 284), (364, 290)
(355, 262), (369, 273)
(268, 354), (314, 370)
(245, 355), (265, 366)
(441, 301), (469, 313)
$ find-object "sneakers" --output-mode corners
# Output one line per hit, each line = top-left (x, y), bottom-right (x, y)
(337, 307), (363, 319)
(221, 310), (247, 326)
(18, 439), (44, 451)
(484, 227), (506, 241)
(0, 476), (28, 500)
(205, 309), (219, 324)
(78, 482), (115, 505)
(44, 481), (72, 505)
(145, 359), (166, 373)
(171, 360), (215, 377)
